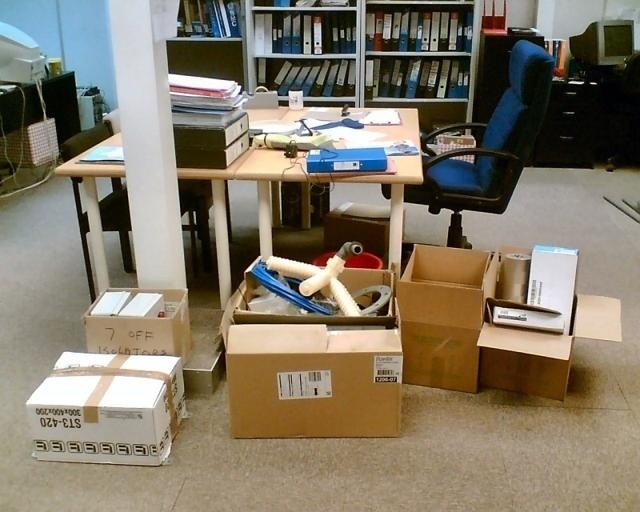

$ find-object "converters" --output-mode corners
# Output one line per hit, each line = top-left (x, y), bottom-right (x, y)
(284, 145), (298, 158)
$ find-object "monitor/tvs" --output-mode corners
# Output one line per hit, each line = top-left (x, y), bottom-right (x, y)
(569, 19), (634, 66)
(0, 21), (45, 85)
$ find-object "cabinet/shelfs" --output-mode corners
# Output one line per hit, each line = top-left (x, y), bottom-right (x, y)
(158, 1), (482, 136)
(470, 32), (600, 173)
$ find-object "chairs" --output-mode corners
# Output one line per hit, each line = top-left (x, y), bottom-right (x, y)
(380, 38), (556, 257)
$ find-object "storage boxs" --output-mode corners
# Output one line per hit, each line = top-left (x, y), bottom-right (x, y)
(220, 241), (404, 434)
(0, 117), (60, 168)
(394, 244), (493, 393)
(475, 245), (579, 401)
(22, 287), (192, 472)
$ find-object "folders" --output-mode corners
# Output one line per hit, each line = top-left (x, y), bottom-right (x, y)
(254, 12), (472, 99)
(307, 148), (387, 173)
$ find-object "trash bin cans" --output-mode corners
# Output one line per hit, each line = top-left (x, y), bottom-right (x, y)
(313, 251), (382, 270)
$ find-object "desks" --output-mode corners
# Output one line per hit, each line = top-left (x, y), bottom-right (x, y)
(1, 69), (81, 163)
(234, 107), (424, 292)
(54, 107), (288, 310)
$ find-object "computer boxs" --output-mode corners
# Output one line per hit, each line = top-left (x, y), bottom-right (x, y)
(77, 94), (104, 131)
